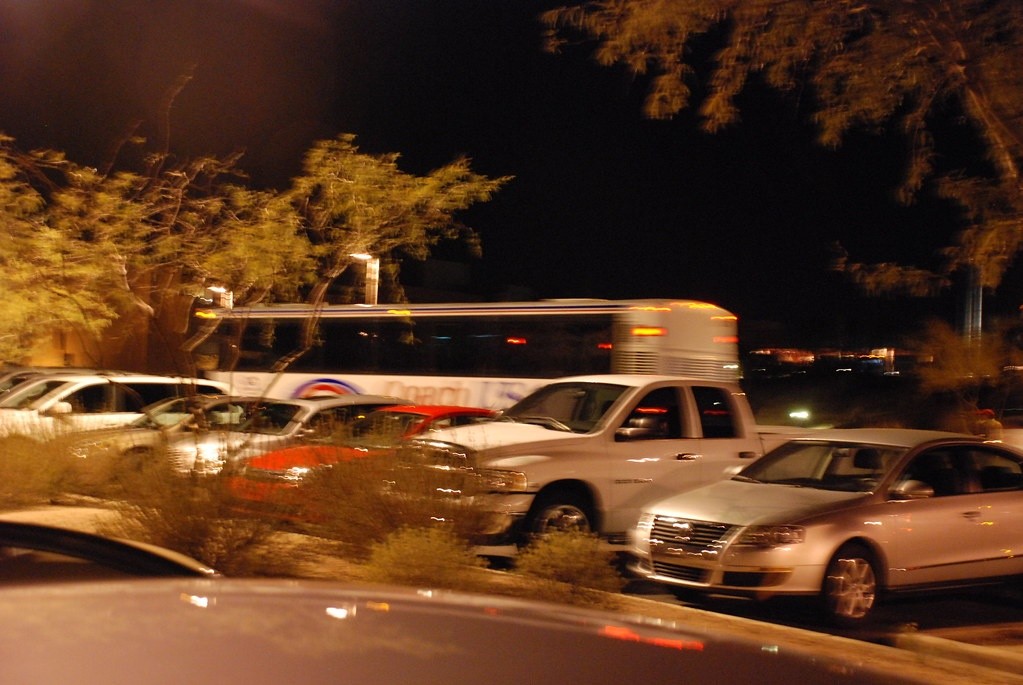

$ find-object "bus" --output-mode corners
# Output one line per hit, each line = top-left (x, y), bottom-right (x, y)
(186, 297), (740, 412)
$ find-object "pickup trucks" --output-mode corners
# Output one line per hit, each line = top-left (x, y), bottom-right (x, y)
(395, 374), (888, 554)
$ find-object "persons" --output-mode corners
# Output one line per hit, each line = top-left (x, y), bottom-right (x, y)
(975, 408), (1006, 441)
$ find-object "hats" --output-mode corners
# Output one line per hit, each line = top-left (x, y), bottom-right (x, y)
(977, 410), (996, 418)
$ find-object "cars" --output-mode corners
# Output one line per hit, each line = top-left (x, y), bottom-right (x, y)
(0, 366), (412, 497)
(624, 429), (1022, 632)
(223, 407), (501, 528)
